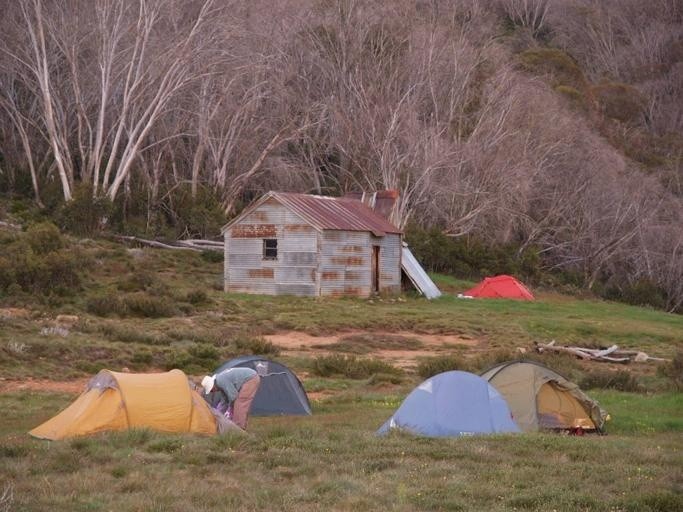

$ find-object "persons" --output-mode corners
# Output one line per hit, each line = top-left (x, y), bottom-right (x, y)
(201, 366), (260, 430)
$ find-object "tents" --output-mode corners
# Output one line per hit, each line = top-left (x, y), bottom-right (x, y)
(27, 369), (246, 441)
(377, 370), (522, 434)
(463, 275), (536, 301)
(204, 356), (312, 418)
(477, 360), (606, 435)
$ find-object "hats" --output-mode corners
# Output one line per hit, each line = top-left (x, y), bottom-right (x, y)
(199, 373), (216, 395)
